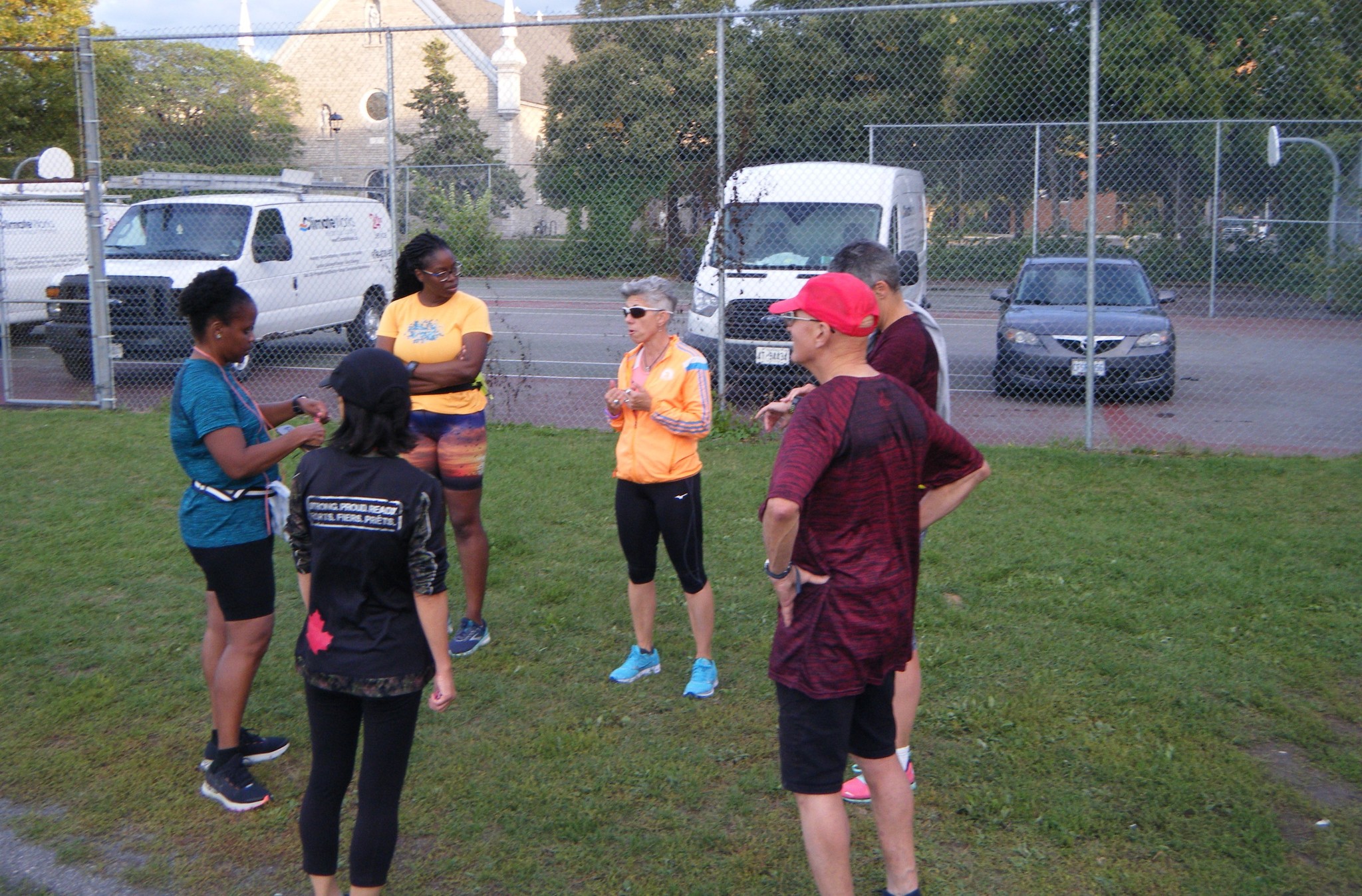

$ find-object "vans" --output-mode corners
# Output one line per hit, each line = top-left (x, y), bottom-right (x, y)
(0, 170), (149, 342)
(43, 191), (402, 386)
(685, 160), (930, 400)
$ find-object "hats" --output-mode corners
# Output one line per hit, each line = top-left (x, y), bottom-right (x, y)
(768, 271), (880, 336)
(318, 347), (411, 409)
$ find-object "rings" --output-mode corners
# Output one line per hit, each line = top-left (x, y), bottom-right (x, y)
(613, 399), (619, 404)
(624, 399), (630, 403)
(626, 388), (631, 393)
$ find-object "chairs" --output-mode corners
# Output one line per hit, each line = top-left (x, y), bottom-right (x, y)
(823, 223), (866, 256)
(745, 222), (799, 263)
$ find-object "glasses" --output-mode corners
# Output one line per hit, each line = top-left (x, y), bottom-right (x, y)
(782, 311), (835, 333)
(622, 306), (674, 318)
(423, 261), (463, 282)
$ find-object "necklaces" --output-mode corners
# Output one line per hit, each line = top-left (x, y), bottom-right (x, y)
(643, 340), (670, 372)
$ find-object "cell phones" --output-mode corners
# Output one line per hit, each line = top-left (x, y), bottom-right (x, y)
(274, 424), (321, 452)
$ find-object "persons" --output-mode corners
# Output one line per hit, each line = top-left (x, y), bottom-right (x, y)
(373, 233), (493, 657)
(169, 266), (331, 811)
(759, 272), (991, 896)
(285, 347), (457, 896)
(603, 274), (719, 699)
(753, 241), (940, 803)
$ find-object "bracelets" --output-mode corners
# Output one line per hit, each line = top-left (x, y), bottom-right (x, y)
(605, 407), (622, 419)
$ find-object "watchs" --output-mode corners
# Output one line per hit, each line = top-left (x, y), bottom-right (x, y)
(405, 360), (419, 379)
(788, 396), (802, 414)
(290, 393), (309, 415)
(764, 558), (793, 579)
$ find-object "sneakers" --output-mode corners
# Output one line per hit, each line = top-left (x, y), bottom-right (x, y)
(683, 658), (719, 697)
(841, 761), (917, 802)
(447, 615), (491, 656)
(201, 759), (271, 810)
(609, 644), (660, 682)
(202, 728), (289, 763)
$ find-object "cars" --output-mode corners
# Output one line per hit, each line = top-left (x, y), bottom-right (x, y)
(989, 258), (1181, 399)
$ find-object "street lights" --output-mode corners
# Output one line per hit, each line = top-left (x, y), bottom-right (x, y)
(324, 111), (343, 189)
